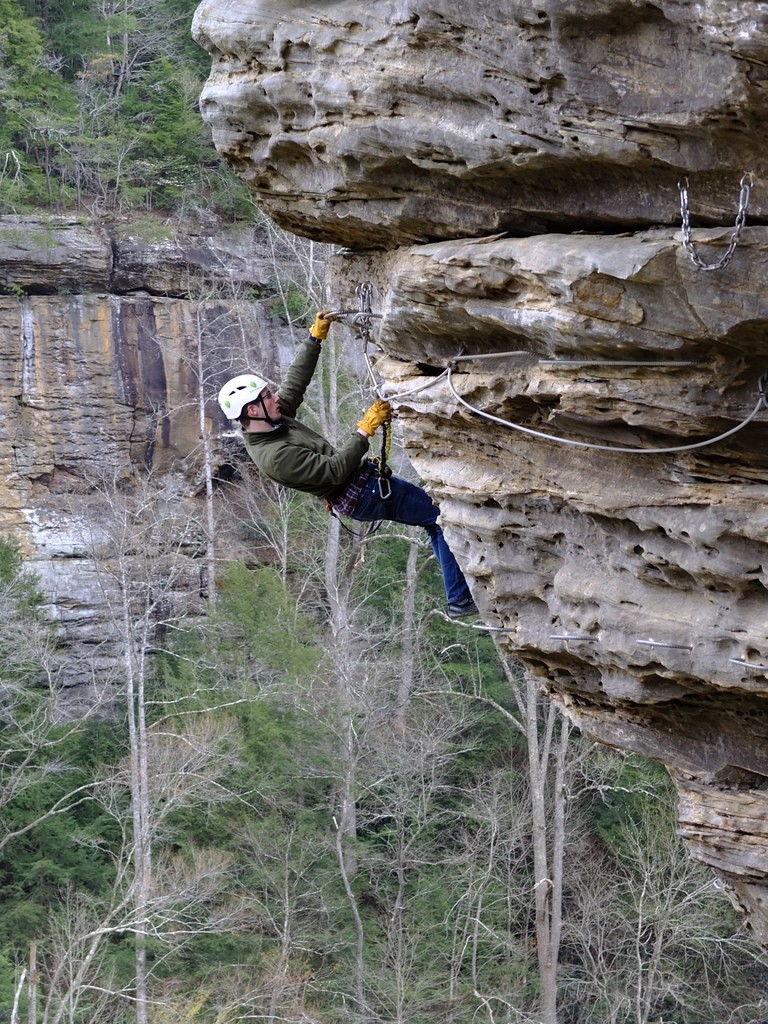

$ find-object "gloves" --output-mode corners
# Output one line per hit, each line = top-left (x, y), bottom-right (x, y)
(356, 397), (392, 437)
(309, 310), (337, 340)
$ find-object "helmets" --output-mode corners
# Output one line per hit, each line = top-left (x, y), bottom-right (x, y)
(218, 374), (269, 419)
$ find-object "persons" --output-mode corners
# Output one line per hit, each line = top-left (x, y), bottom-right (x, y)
(217, 308), (480, 619)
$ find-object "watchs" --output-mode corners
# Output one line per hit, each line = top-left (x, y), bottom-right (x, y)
(306, 332), (322, 344)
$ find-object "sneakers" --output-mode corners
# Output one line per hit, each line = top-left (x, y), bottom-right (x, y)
(448, 597), (480, 617)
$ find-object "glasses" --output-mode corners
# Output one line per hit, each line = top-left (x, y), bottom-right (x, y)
(246, 391), (271, 415)
